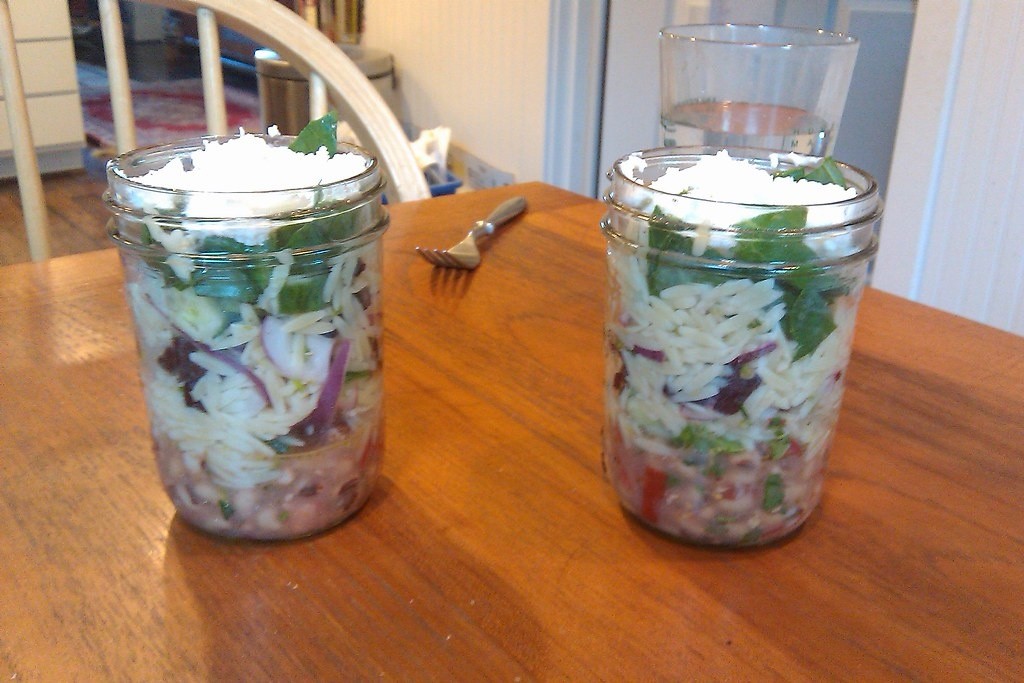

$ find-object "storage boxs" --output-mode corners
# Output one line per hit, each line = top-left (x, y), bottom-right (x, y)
(381, 166), (463, 204)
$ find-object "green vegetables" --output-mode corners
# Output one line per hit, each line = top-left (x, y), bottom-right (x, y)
(644, 155), (846, 363)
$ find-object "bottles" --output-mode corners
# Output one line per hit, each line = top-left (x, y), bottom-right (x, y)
(103, 133), (390, 539)
(604, 150), (880, 545)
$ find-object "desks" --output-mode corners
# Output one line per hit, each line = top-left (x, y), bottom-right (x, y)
(0, 179), (1024, 683)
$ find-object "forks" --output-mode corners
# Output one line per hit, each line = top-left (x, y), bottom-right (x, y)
(414, 195), (527, 270)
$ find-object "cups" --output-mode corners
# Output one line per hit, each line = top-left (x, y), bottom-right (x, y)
(657, 22), (861, 159)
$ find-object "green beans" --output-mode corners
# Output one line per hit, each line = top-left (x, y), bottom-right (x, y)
(139, 109), (355, 310)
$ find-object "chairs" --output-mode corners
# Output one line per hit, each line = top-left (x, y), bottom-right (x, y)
(0, 0), (431, 263)
